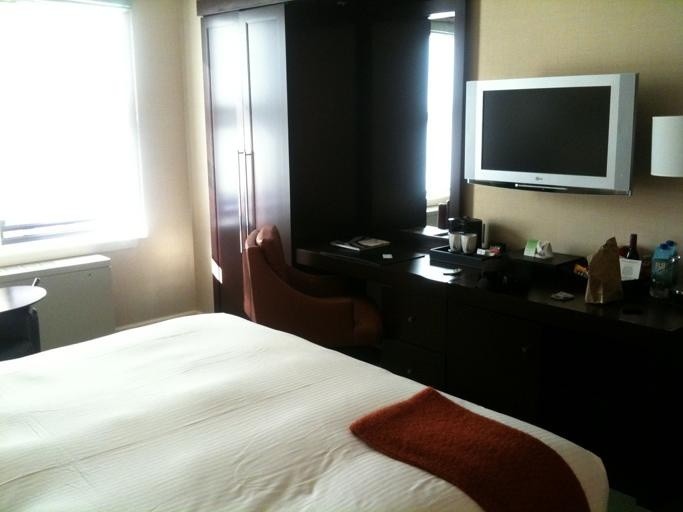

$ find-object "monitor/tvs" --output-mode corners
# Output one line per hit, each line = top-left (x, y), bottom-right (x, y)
(464, 72), (640, 197)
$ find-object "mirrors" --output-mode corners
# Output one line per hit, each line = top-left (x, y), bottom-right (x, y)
(361, 1), (467, 248)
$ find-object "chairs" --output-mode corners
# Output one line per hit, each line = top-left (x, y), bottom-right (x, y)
(239, 223), (383, 346)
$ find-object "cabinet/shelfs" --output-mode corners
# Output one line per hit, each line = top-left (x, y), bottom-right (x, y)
(195, 0), (360, 328)
(359, 14), (431, 225)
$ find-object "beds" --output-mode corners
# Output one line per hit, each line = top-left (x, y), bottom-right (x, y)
(1, 312), (610, 512)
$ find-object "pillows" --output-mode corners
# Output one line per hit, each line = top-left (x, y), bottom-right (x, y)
(255, 223), (285, 283)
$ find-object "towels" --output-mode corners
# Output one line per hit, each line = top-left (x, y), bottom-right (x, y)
(348, 387), (591, 512)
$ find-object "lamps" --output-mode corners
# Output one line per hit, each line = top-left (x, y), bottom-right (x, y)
(650, 116), (682, 179)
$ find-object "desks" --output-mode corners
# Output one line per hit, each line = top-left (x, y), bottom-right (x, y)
(296, 222), (683, 512)
(1, 286), (47, 360)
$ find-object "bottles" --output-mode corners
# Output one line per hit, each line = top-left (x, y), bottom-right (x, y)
(625, 233), (641, 290)
(650, 239), (678, 300)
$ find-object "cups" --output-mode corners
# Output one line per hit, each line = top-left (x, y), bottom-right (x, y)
(447, 230), (478, 256)
